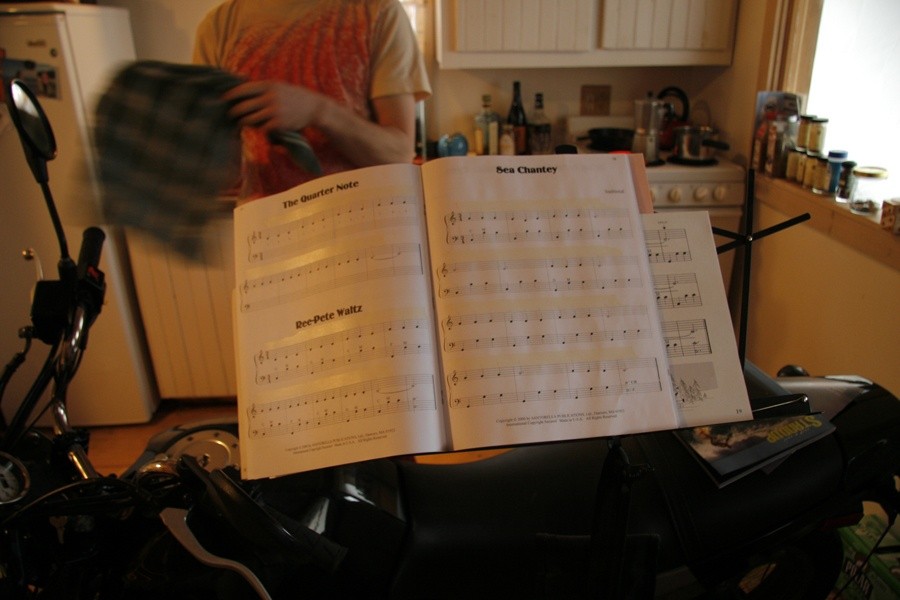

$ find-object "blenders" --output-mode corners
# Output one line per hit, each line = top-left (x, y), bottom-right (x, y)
(631, 98), (660, 162)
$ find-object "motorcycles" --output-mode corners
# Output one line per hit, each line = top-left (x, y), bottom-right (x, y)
(1, 75), (900, 600)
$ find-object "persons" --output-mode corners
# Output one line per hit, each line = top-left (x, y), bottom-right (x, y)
(192, 0), (433, 208)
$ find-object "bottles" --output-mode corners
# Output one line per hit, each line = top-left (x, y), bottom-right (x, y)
(849, 167), (889, 215)
(506, 80), (528, 155)
(500, 124), (516, 156)
(785, 115), (857, 198)
(473, 94), (498, 156)
(527, 92), (551, 155)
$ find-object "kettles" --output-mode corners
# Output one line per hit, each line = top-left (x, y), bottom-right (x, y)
(647, 86), (691, 151)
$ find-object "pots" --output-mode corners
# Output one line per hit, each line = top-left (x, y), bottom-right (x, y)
(671, 125), (730, 160)
(588, 127), (634, 153)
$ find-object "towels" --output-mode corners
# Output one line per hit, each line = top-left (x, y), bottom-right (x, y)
(94, 59), (247, 263)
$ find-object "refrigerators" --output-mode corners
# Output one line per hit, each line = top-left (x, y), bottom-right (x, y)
(0, 4), (159, 426)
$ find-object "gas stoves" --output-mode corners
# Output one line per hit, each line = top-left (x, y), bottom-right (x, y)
(573, 133), (746, 205)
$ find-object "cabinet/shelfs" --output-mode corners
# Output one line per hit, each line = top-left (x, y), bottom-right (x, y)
(435, 0), (739, 71)
(124, 220), (237, 397)
